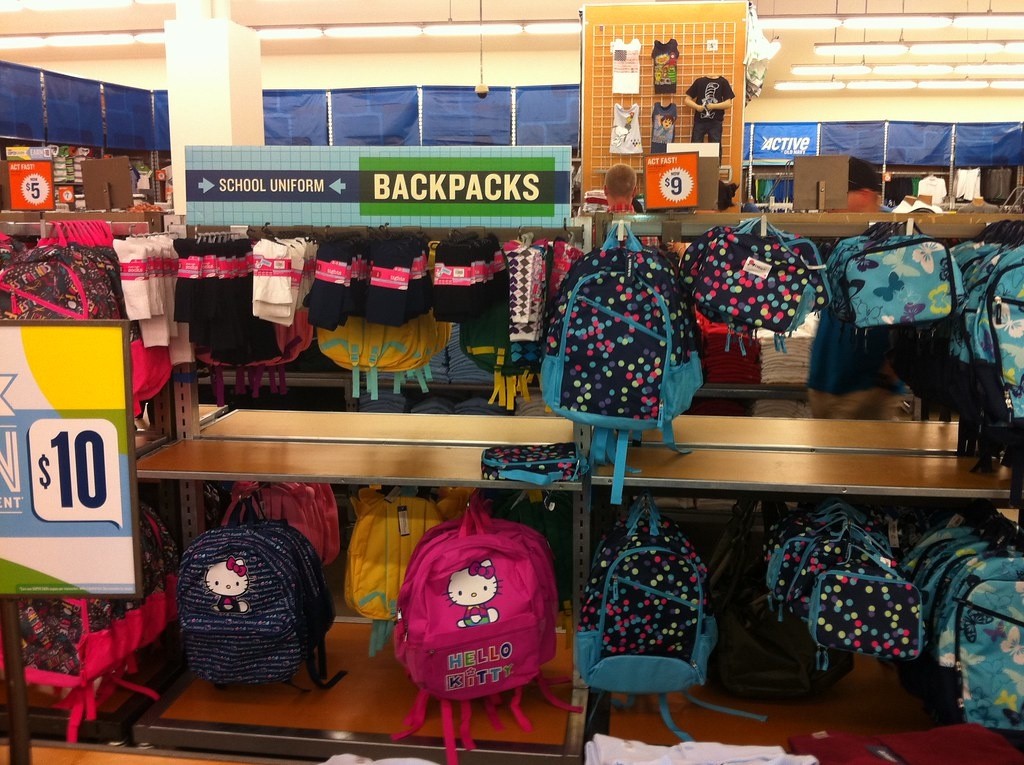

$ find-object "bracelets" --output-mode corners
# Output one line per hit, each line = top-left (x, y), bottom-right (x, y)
(677, 246), (684, 256)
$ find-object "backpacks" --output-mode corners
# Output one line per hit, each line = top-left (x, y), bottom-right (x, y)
(0, 221), (171, 416)
(459, 221), (1024, 505)
(190, 240), (452, 407)
(0, 481), (1024, 765)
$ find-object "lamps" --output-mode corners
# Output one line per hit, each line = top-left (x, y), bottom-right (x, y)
(474, 0), (490, 99)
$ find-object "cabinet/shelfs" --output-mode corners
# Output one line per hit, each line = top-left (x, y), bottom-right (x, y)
(586, 211), (1024, 765)
(0, 220), (176, 748)
(131, 223), (582, 765)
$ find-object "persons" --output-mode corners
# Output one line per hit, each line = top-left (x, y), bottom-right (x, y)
(664, 179), (738, 267)
(683, 75), (736, 168)
(802, 159), (909, 423)
(593, 164), (661, 249)
